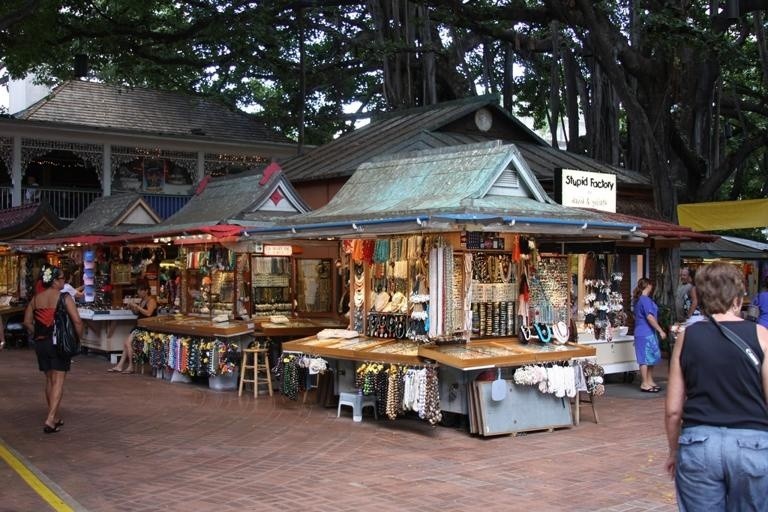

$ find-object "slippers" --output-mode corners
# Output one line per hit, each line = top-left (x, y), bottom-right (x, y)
(106, 368), (122, 372)
(641, 386), (660, 393)
(123, 370), (134, 374)
(43, 420), (63, 432)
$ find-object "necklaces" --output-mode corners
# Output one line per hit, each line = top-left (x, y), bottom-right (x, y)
(499, 262), (512, 284)
(268, 353), (443, 428)
(521, 325), (531, 341)
(533, 323), (552, 343)
(350, 236), (472, 348)
(130, 328), (239, 379)
(556, 323), (569, 338)
(487, 254), (503, 284)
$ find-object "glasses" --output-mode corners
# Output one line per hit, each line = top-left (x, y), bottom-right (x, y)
(55, 275), (65, 280)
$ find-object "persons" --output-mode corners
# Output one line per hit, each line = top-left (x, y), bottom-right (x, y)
(633, 277), (669, 393)
(674, 265), (701, 323)
(749, 276), (768, 329)
(23, 262), (84, 433)
(59, 270), (84, 303)
(106, 278), (158, 374)
(664, 261), (768, 512)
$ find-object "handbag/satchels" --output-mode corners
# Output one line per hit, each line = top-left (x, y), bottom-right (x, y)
(55, 309), (79, 361)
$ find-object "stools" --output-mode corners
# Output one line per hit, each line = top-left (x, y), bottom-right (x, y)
(238, 347), (273, 398)
(337, 392), (378, 422)
